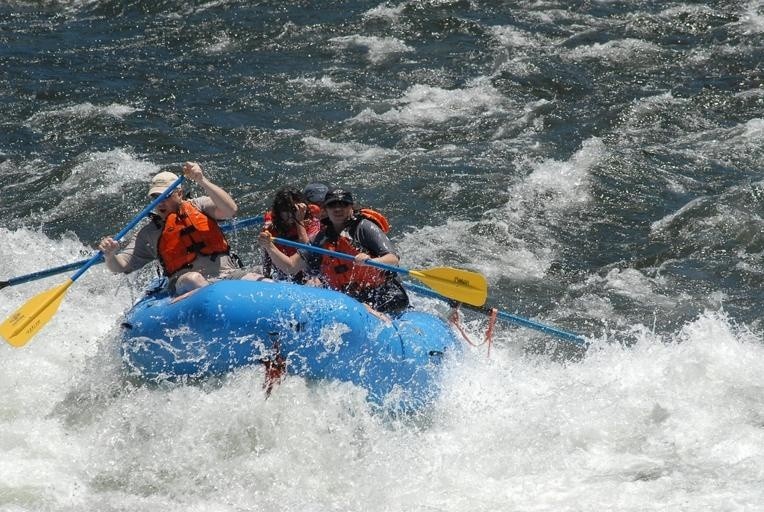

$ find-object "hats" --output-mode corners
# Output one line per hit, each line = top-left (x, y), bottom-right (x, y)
(302, 182), (353, 206)
(148, 171), (181, 196)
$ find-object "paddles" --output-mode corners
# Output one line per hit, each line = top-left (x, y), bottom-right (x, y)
(0, 175), (186, 347)
(264, 230), (488, 307)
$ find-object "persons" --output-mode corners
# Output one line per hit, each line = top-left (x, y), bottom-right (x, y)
(263, 185), (321, 285)
(97, 160), (275, 305)
(302, 182), (330, 220)
(255, 186), (410, 314)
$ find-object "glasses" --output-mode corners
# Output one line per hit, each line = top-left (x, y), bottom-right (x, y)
(327, 201), (348, 209)
(151, 190), (173, 201)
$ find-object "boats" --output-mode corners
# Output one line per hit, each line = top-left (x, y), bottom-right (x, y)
(117, 276), (457, 435)
